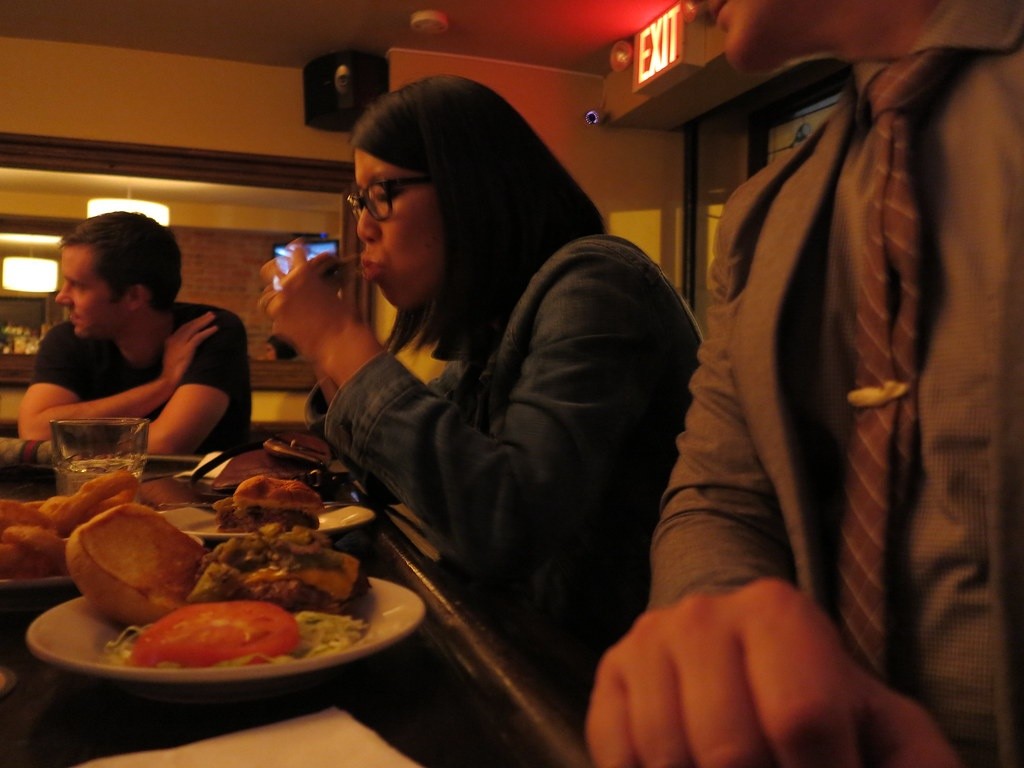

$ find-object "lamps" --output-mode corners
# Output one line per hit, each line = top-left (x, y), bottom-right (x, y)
(2, 243), (58, 293)
(86, 185), (171, 229)
(611, 0), (715, 94)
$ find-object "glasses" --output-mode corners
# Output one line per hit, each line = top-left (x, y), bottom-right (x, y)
(346, 176), (430, 220)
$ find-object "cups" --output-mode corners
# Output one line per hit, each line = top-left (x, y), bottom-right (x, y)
(49, 415), (150, 496)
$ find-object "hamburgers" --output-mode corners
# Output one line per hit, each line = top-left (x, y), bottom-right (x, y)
(64, 502), (372, 629)
(213, 474), (324, 533)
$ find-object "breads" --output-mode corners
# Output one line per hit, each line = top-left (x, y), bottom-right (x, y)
(0, 470), (137, 587)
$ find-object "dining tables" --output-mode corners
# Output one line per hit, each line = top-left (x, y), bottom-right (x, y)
(0, 453), (593, 768)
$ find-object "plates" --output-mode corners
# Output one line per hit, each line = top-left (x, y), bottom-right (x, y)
(0, 531), (205, 612)
(25, 577), (430, 705)
(157, 503), (377, 547)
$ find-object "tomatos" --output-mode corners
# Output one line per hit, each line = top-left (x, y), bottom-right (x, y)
(133, 598), (301, 669)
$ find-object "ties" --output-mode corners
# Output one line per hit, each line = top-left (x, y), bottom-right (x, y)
(837, 46), (954, 684)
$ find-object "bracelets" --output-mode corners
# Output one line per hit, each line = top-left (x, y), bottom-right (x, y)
(20, 440), (45, 462)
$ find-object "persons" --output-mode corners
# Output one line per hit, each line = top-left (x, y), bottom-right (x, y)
(588, 0), (1023, 768)
(0, 438), (54, 469)
(19, 212), (251, 457)
(262, 77), (704, 721)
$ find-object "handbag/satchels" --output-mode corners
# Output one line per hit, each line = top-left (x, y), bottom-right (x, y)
(191, 432), (332, 500)
